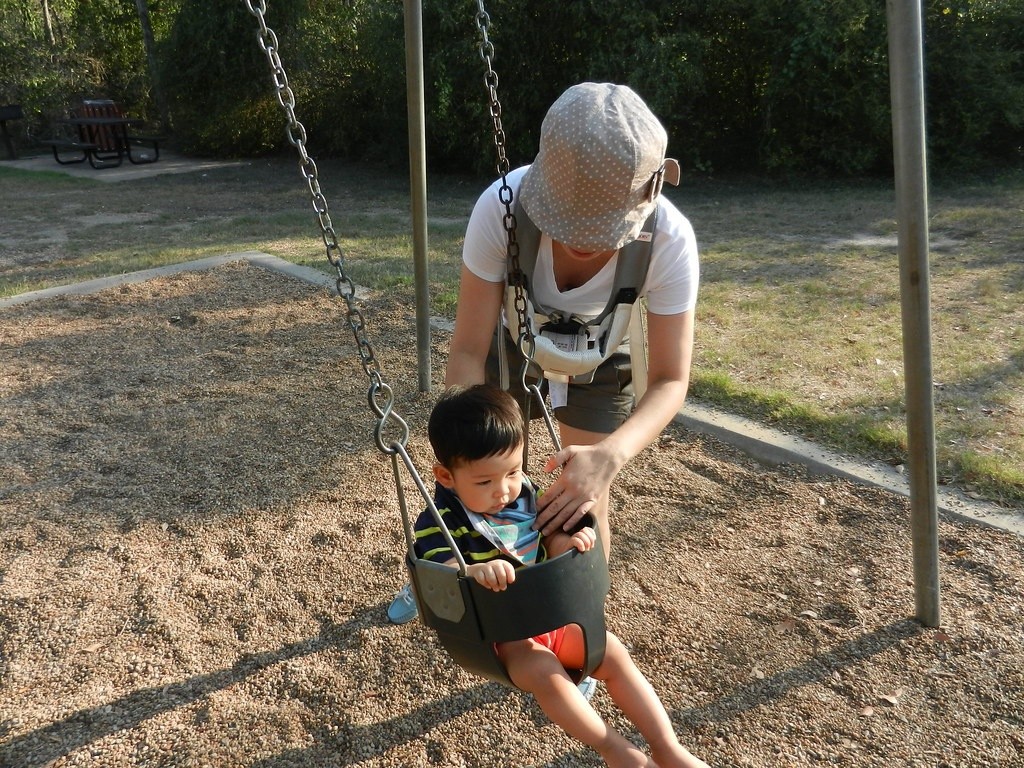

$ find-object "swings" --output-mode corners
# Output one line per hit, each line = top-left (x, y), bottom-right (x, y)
(245, 0), (610, 686)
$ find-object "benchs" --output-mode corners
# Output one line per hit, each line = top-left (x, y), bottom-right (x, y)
(118, 135), (160, 164)
(41, 136), (100, 170)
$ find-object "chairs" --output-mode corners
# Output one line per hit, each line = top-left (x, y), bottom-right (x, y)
(401, 509), (614, 698)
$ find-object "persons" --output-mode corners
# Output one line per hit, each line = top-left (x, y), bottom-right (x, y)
(411, 382), (712, 768)
(387, 82), (701, 705)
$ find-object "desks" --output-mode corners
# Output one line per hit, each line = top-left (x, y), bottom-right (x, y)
(63, 116), (144, 167)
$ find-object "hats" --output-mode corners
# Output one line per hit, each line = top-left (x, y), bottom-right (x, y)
(518, 82), (680, 250)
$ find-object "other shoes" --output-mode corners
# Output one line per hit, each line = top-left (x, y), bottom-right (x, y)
(387, 583), (420, 624)
(576, 675), (596, 702)
